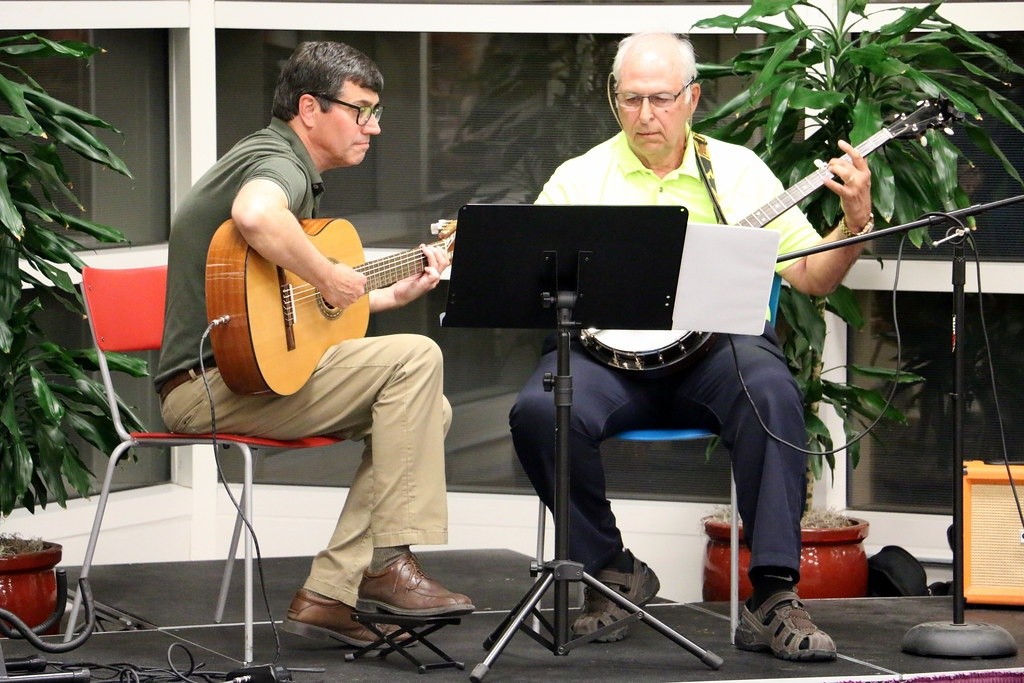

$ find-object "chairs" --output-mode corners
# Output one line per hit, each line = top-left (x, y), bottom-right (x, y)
(62, 266), (346, 665)
(532, 269), (785, 636)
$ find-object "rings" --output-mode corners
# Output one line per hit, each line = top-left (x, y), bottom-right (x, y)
(845, 175), (853, 185)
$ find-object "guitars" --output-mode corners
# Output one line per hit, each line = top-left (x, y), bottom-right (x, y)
(580, 90), (966, 374)
(204, 216), (455, 400)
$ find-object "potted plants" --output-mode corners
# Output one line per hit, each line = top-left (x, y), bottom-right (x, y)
(0, 29), (151, 641)
(684, 0), (1024, 602)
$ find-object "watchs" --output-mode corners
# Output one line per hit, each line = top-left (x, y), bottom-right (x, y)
(839, 213), (874, 238)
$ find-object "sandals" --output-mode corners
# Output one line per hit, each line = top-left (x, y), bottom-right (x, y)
(735, 590), (838, 661)
(571, 549), (660, 641)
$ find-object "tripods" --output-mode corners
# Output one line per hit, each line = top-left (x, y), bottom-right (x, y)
(440, 203), (725, 683)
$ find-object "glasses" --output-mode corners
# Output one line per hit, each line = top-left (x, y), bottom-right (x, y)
(615, 80), (694, 111)
(312, 92), (383, 126)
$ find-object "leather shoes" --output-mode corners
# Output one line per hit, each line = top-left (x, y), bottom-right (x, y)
(279, 588), (419, 648)
(357, 550), (476, 614)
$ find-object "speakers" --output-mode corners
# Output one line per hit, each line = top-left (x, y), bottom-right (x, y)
(961, 459), (1024, 608)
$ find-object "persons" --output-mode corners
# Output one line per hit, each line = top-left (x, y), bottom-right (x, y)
(507, 32), (872, 661)
(151, 40), (476, 648)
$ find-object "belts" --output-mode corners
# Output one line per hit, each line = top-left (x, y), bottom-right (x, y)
(159, 358), (216, 400)
